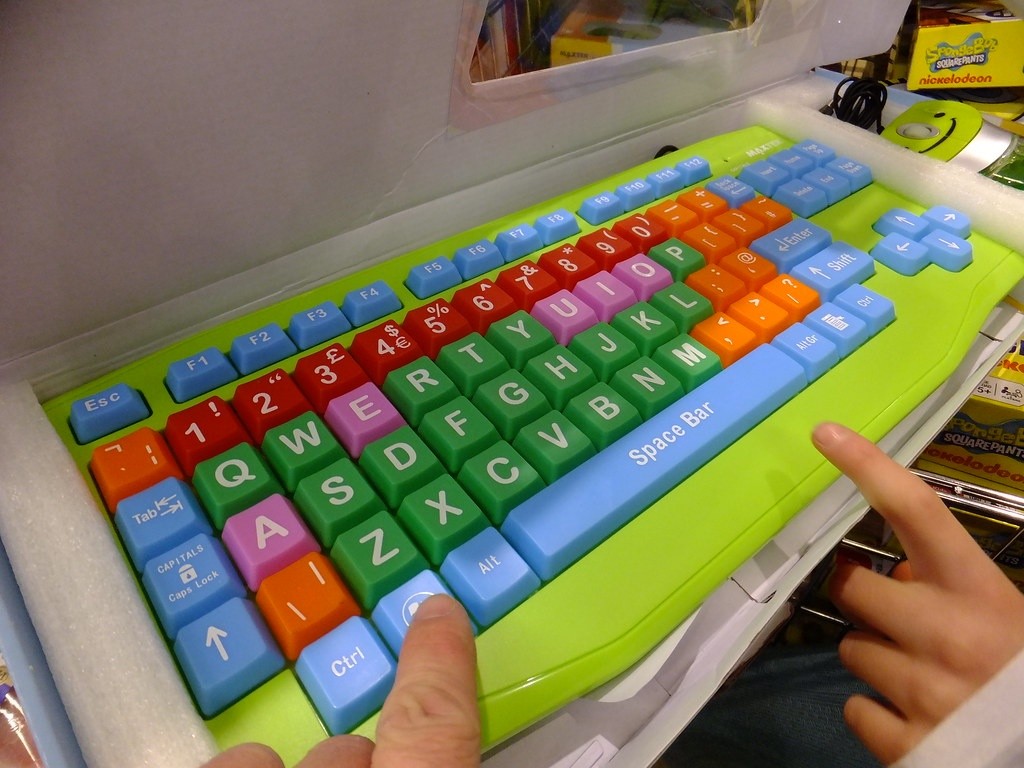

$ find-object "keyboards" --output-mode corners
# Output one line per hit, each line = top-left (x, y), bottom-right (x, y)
(36, 123), (1024, 768)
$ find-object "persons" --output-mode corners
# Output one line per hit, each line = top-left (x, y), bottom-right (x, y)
(191, 594), (482, 768)
(810, 420), (1024, 768)
